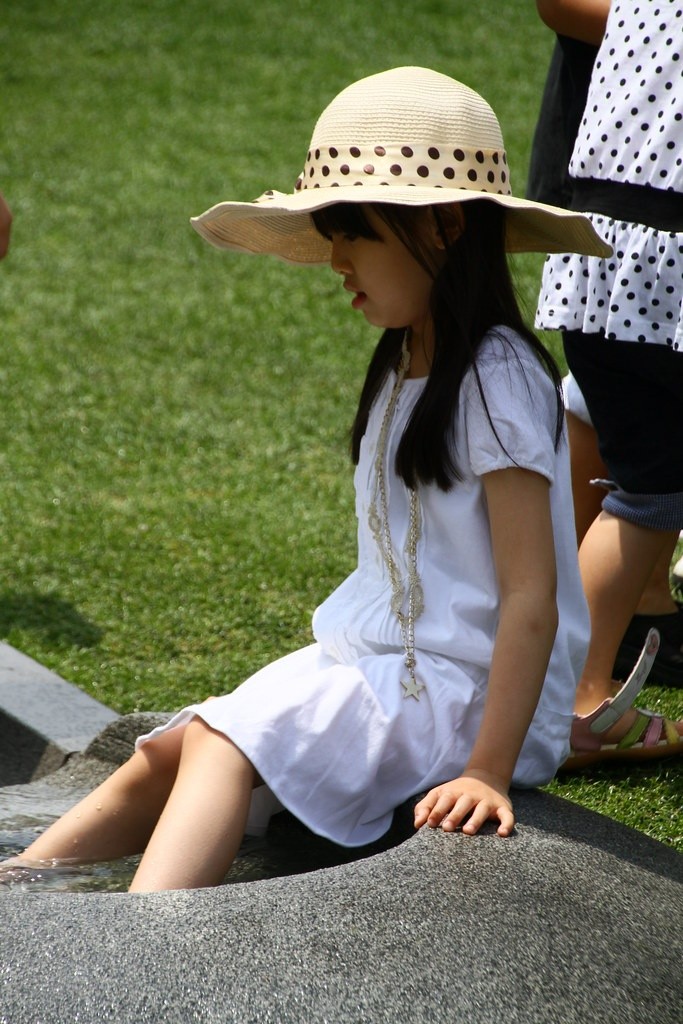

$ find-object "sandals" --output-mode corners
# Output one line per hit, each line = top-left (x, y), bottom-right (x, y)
(562, 628), (683, 770)
(190, 66), (614, 266)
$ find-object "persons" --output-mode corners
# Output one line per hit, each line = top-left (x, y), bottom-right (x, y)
(524, 1), (683, 763)
(12, 63), (611, 893)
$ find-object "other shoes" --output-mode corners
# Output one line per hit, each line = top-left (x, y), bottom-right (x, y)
(611, 601), (683, 686)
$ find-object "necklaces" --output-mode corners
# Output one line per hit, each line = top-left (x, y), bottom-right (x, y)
(367, 330), (425, 702)
